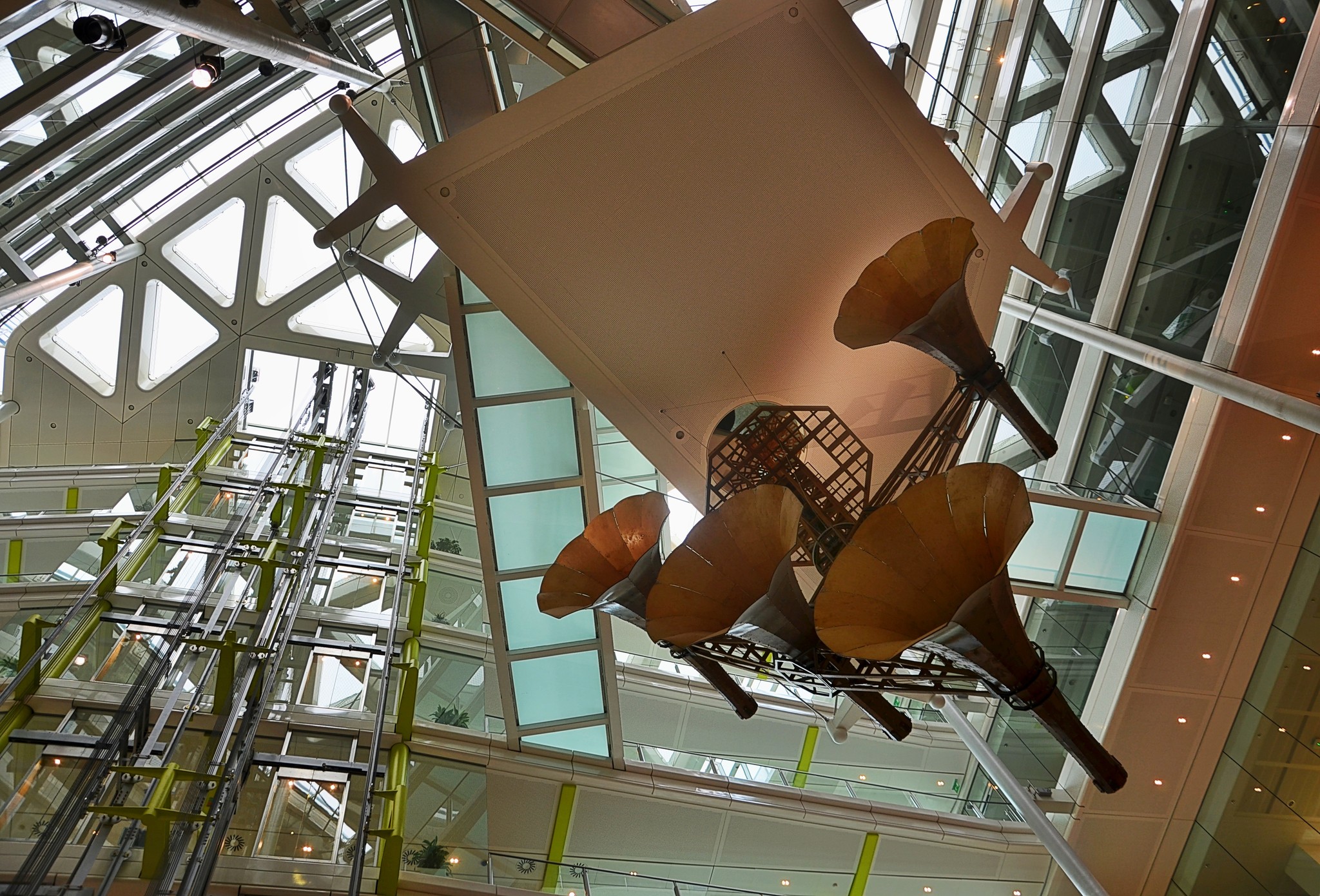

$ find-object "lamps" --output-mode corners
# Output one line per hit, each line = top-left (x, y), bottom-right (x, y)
(337, 80), (350, 90)
(346, 89), (357, 102)
(258, 57), (279, 77)
(73, 15), (128, 53)
(309, 17), (332, 33)
(192, 38), (225, 87)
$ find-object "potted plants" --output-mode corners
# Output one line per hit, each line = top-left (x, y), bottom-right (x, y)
(412, 836), (453, 878)
